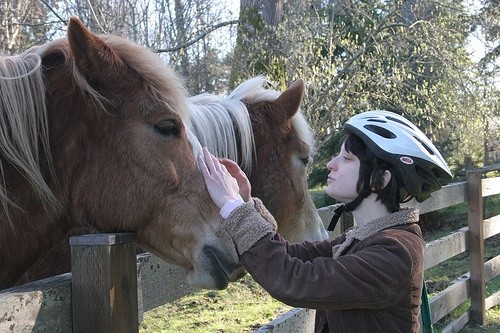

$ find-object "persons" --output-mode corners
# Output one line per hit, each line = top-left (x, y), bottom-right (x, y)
(197, 110), (453, 333)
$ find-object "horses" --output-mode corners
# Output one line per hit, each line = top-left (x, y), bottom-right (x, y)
(0, 15), (329, 333)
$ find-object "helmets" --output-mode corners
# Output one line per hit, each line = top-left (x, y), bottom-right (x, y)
(344, 110), (453, 203)
